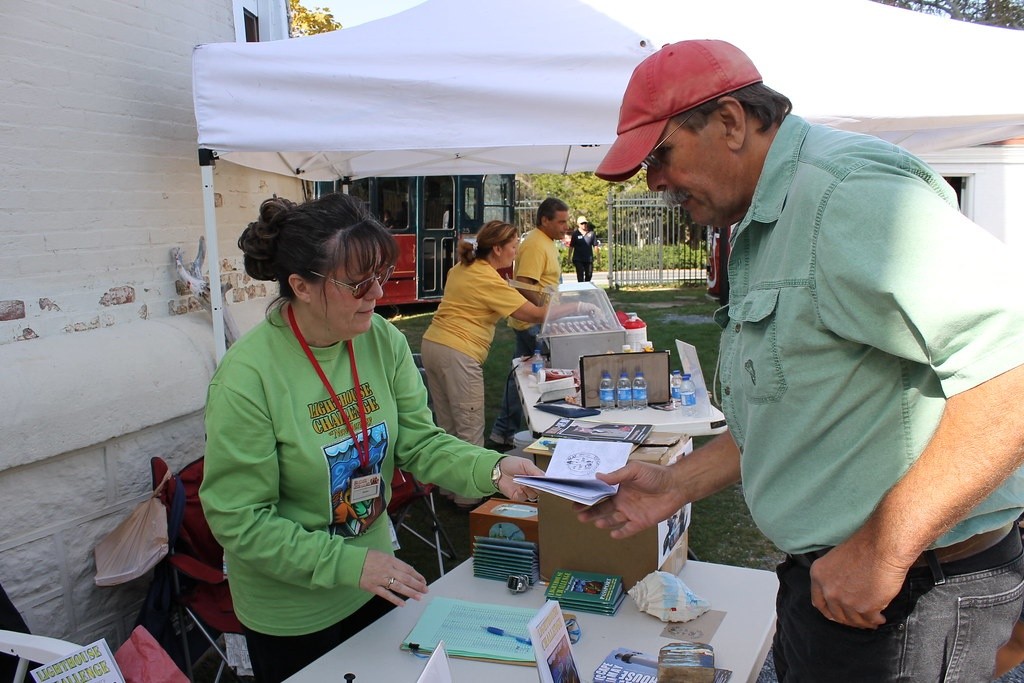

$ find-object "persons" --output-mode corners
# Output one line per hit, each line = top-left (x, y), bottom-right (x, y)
(572, 39), (1024, 683)
(199, 195), (547, 683)
(421, 220), (601, 508)
(567, 215), (599, 282)
(489, 197), (570, 448)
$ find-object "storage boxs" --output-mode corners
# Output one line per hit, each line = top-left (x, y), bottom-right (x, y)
(536, 433), (694, 590)
(469, 497), (539, 558)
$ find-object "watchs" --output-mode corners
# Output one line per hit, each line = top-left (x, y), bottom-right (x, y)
(491, 461), (502, 489)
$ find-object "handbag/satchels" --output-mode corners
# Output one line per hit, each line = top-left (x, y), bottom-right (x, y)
(95, 469), (172, 586)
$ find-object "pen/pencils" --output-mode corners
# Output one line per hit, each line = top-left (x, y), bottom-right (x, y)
(539, 582), (550, 586)
(480, 625), (532, 646)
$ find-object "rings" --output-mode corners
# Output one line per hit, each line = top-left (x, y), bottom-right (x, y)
(386, 577), (394, 589)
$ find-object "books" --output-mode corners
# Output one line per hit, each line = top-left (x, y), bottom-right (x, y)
(542, 417), (655, 444)
(512, 439), (633, 506)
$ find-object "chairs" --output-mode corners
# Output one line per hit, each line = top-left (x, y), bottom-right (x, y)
(147, 446), (460, 683)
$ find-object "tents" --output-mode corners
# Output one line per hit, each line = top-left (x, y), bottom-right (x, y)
(191, 0), (1024, 367)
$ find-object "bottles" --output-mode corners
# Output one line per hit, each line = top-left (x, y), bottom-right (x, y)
(671, 370), (682, 409)
(621, 341), (654, 353)
(633, 373), (648, 409)
(617, 373), (632, 409)
(532, 350), (543, 381)
(598, 373), (615, 411)
(684, 374), (696, 394)
(680, 377), (696, 417)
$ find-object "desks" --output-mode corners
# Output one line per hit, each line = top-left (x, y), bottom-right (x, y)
(511, 354), (726, 436)
(283, 557), (782, 683)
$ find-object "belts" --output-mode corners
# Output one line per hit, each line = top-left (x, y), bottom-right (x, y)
(792, 521), (1014, 568)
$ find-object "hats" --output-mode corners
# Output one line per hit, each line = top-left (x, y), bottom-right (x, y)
(595, 40), (762, 182)
(577, 215), (589, 223)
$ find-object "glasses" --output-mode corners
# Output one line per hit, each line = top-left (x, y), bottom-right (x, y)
(640, 96), (717, 171)
(307, 264), (395, 299)
